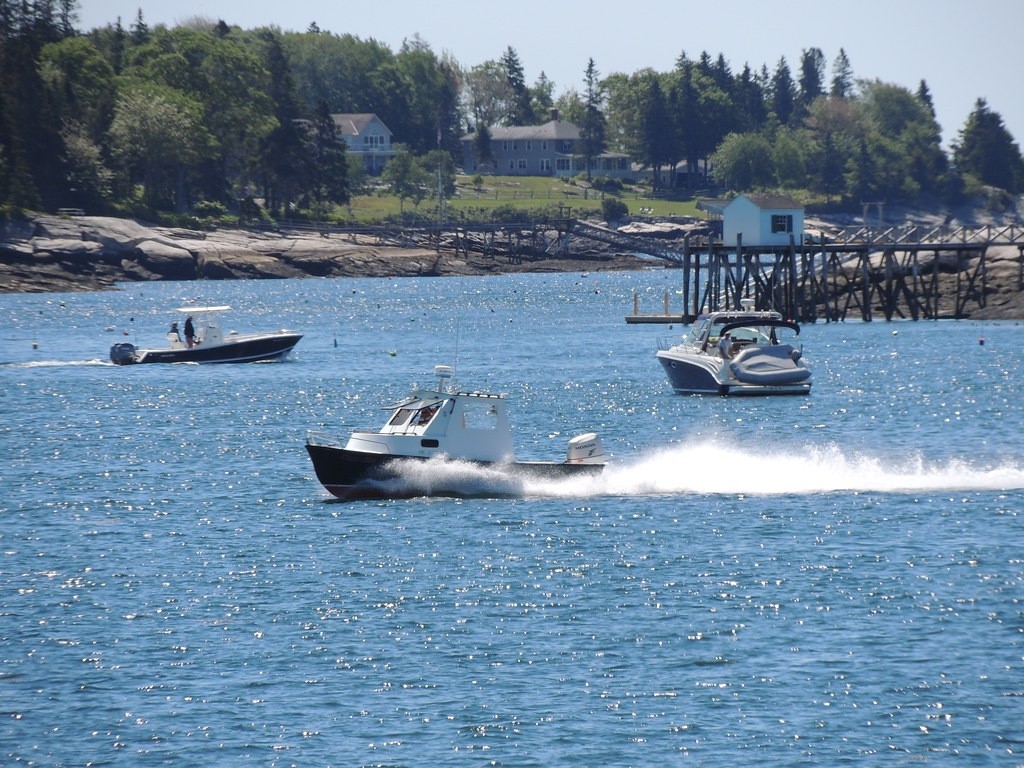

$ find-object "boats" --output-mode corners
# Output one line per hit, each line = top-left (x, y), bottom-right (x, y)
(110, 306), (303, 365)
(655, 297), (815, 397)
(305, 365), (606, 504)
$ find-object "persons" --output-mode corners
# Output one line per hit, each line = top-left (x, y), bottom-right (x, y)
(183, 316), (196, 349)
(167, 321), (182, 343)
(719, 331), (735, 360)
(418, 405), (433, 426)
